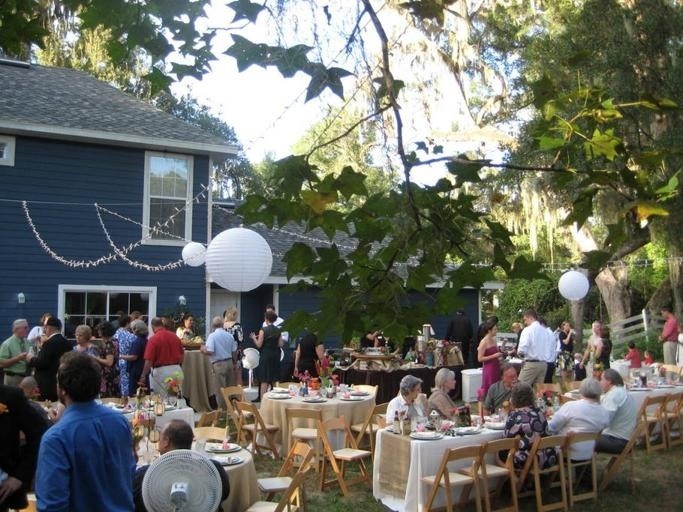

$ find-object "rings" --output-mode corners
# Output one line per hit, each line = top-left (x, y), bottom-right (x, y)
(3, 490), (9, 496)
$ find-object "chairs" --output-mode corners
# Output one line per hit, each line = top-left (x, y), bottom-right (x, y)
(315, 415), (373, 496)
(40, 395), (231, 443)
(421, 441), (485, 512)
(513, 431), (569, 512)
(220, 385), (248, 441)
(233, 399), (280, 460)
(546, 431), (597, 507)
(531, 363), (682, 453)
(349, 401), (388, 464)
(597, 410), (642, 494)
(459, 434), (519, 512)
(244, 463), (311, 512)
(284, 406), (323, 473)
(256, 439), (313, 512)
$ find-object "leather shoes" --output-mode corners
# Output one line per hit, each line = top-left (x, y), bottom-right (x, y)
(252, 399), (260, 402)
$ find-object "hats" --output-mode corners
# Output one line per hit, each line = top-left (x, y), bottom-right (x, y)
(478, 315), (498, 338)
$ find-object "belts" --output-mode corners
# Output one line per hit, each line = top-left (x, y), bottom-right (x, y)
(213, 358), (232, 363)
(666, 339), (677, 341)
(153, 364), (179, 368)
(526, 360), (539, 362)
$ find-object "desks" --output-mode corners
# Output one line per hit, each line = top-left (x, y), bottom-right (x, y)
(375, 415), (504, 512)
(137, 441), (251, 482)
(317, 351), (469, 404)
(261, 381), (379, 457)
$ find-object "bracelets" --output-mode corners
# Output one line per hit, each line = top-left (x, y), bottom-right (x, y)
(503, 350), (509, 355)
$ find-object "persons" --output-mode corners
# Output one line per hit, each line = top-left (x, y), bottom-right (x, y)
(468, 304), (682, 388)
(475, 316), (516, 422)
(249, 303), (292, 403)
(26, 312), (55, 362)
(385, 374), (426, 426)
(293, 326), (332, 383)
(132, 419), (230, 511)
(34, 350), (137, 512)
(73, 305), (245, 397)
(548, 377), (610, 482)
(595, 369), (638, 455)
(0, 374), (46, 512)
(357, 330), (401, 357)
(446, 307), (473, 401)
(426, 367), (471, 420)
(31, 316), (73, 397)
(495, 381), (556, 486)
(483, 362), (534, 415)
(1, 318), (38, 389)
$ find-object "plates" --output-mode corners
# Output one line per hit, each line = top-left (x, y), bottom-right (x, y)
(409, 421), (504, 440)
(268, 386), (369, 402)
(627, 384), (675, 391)
(201, 441), (244, 465)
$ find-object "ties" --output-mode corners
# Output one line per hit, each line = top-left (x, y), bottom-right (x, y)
(20, 341), (26, 353)
